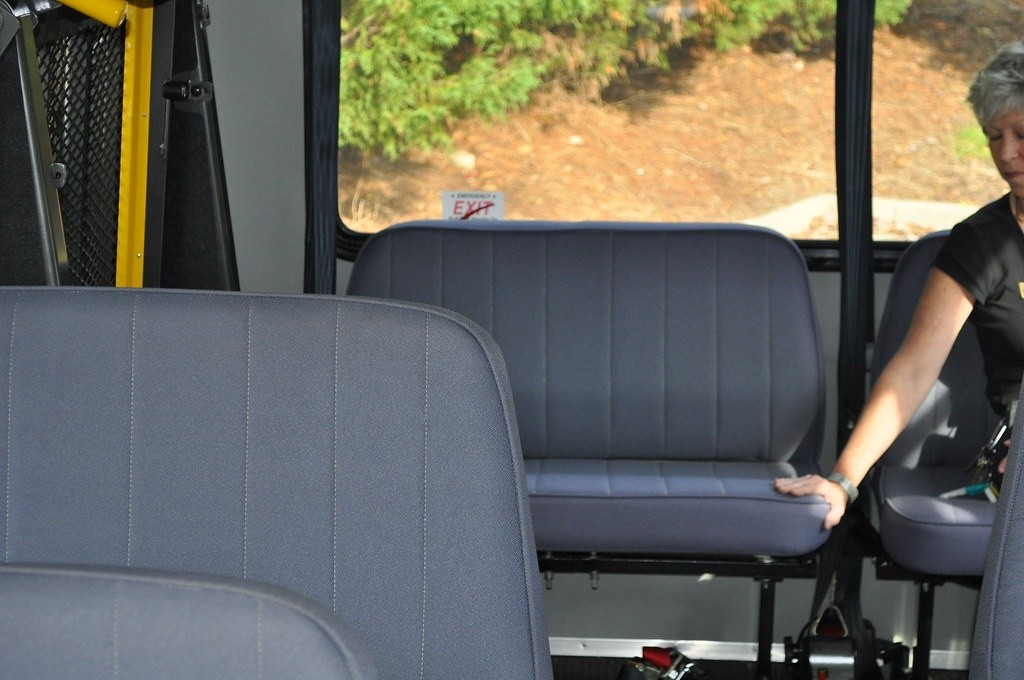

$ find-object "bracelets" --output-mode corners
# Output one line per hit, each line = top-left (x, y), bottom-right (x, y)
(827, 473), (859, 503)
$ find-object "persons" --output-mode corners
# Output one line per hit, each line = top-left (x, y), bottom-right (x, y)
(773, 45), (1023, 533)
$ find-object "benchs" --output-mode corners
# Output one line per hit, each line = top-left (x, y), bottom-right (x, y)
(0, 284), (554, 680)
(0, 562), (377, 679)
(967, 378), (1024, 680)
(869, 229), (998, 680)
(345, 220), (832, 680)
